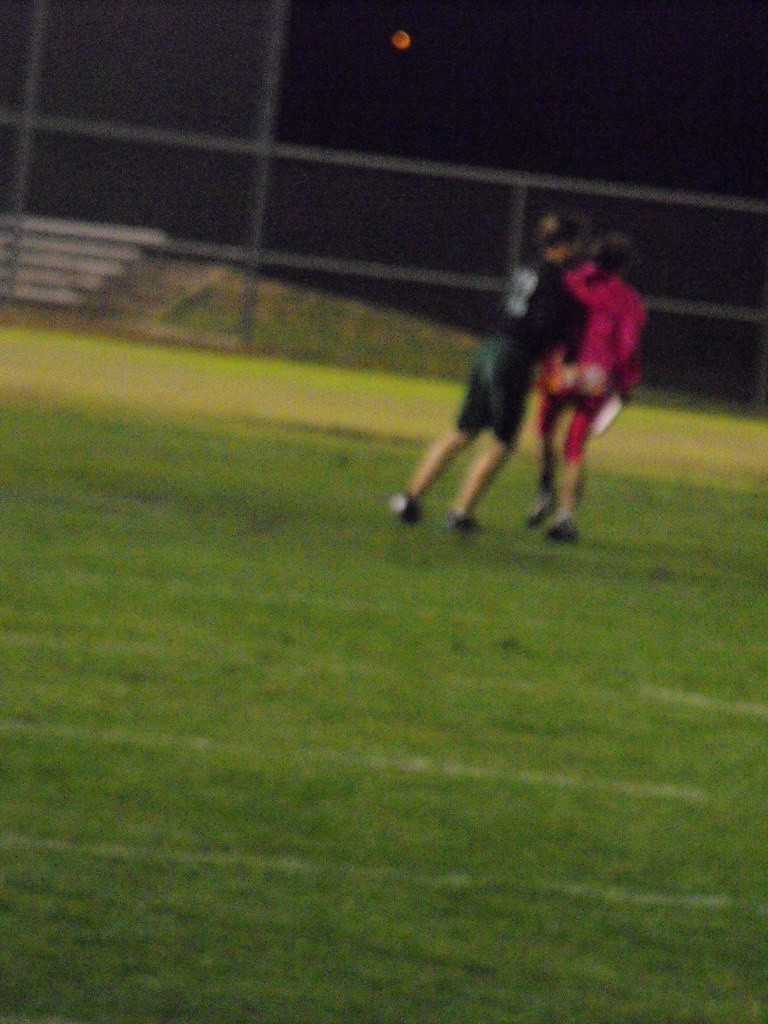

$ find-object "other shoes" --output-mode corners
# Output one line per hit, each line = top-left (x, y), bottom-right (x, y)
(547, 518), (580, 544)
(392, 489), (423, 522)
(444, 508), (477, 533)
(528, 495), (557, 526)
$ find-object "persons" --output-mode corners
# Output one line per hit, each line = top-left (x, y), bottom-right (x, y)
(526, 229), (646, 547)
(388, 211), (588, 536)
